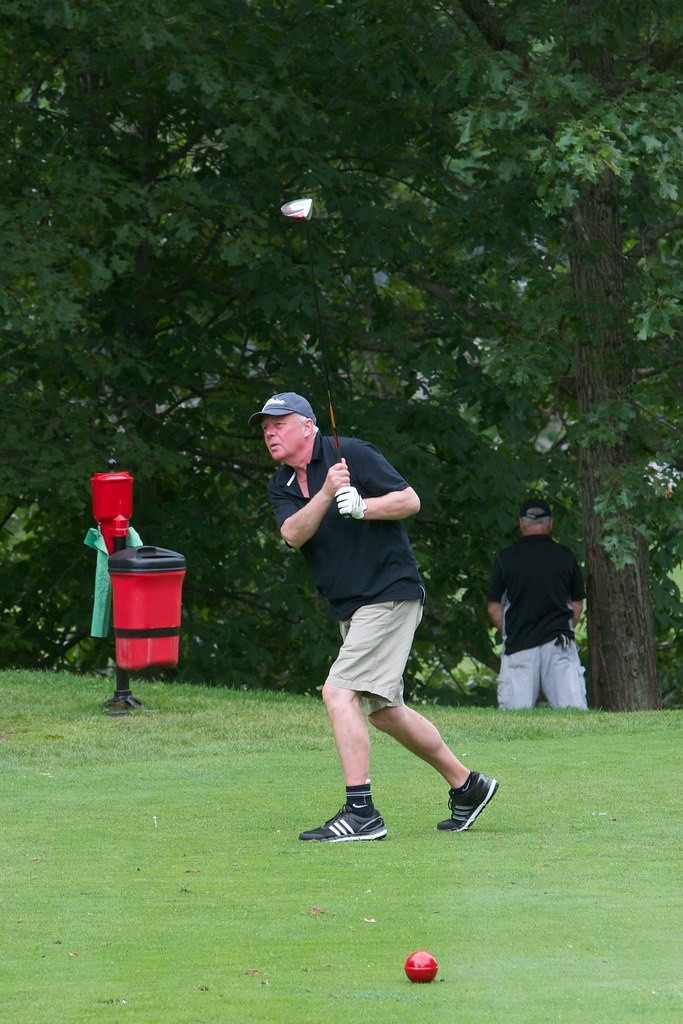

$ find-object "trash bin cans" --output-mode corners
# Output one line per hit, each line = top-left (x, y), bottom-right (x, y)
(107, 545), (186, 671)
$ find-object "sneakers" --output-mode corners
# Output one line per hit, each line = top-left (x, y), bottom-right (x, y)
(298, 806), (387, 841)
(437, 775), (498, 832)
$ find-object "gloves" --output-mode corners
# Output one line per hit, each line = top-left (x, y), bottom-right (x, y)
(554, 634), (570, 650)
(335, 486), (368, 519)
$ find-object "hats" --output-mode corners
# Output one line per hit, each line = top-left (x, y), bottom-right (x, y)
(519, 498), (552, 519)
(248, 392), (316, 426)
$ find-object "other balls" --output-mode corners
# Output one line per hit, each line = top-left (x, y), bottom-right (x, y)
(403, 950), (439, 983)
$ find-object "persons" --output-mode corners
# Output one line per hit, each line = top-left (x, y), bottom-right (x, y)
(487, 498), (586, 711)
(247, 392), (499, 843)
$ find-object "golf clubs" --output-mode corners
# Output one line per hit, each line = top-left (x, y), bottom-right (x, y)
(278, 197), (352, 521)
